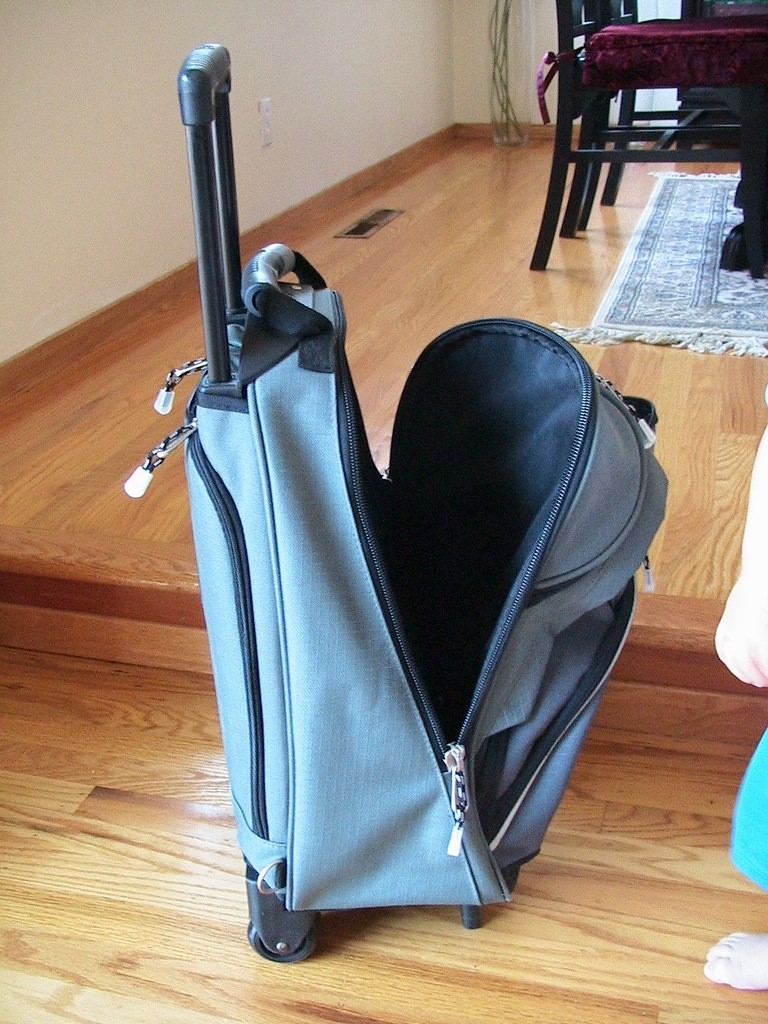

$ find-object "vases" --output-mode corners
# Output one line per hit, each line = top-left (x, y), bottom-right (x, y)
(485, 0), (537, 147)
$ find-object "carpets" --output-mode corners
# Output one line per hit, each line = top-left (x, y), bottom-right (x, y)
(549, 170), (768, 359)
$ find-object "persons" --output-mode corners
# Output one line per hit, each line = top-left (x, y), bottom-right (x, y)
(704, 384), (768, 989)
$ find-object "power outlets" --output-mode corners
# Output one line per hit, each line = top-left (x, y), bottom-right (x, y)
(259, 97), (273, 147)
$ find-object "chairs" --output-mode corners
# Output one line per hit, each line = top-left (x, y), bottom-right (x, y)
(527, 0), (739, 272)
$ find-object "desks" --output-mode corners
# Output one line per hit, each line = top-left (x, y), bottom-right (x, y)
(584, 18), (768, 273)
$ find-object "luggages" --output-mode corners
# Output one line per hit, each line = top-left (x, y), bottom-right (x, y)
(124, 43), (670, 965)
